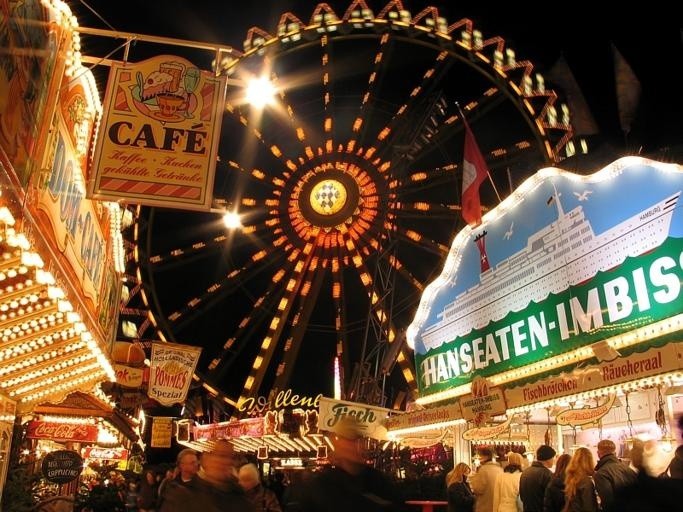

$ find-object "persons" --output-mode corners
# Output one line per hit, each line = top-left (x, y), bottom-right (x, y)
(278, 417), (408, 511)
(157, 438), (278, 512)
(445, 439), (682, 511)
(78, 470), (162, 511)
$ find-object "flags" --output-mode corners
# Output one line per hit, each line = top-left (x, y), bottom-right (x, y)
(461, 130), (486, 227)
